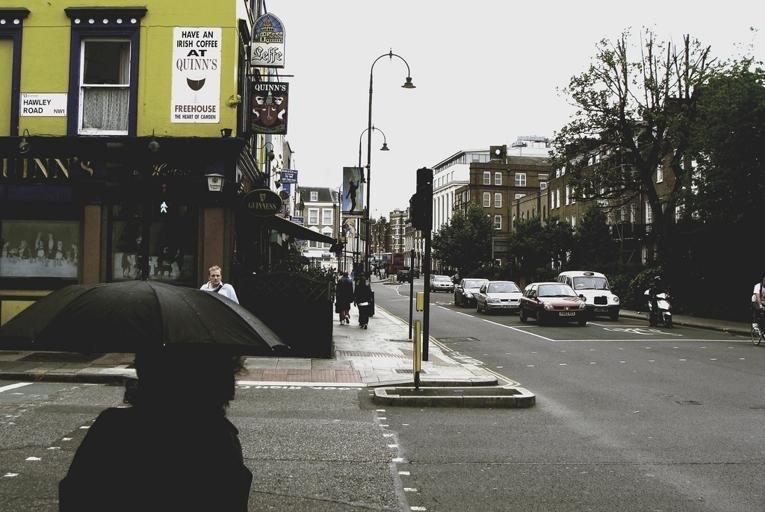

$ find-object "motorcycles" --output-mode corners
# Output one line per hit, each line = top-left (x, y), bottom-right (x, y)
(644, 288), (673, 329)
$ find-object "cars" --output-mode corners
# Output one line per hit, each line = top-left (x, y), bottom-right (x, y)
(519, 282), (588, 328)
(475, 280), (523, 314)
(368, 252), (420, 284)
(429, 274), (455, 293)
(454, 278), (490, 308)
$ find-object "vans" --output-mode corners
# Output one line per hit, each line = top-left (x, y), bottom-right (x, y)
(556, 270), (620, 321)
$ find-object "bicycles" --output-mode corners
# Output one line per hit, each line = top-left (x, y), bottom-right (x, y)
(750, 305), (765, 345)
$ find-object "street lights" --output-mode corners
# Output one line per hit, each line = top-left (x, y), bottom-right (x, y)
(355, 124), (390, 290)
(365, 47), (416, 315)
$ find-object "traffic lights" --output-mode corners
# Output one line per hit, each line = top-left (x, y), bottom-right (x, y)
(151, 179), (173, 220)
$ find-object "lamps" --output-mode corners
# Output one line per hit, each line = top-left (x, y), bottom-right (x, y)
(18, 127), (32, 155)
(148, 128), (161, 154)
(250, 142), (275, 161)
(204, 171), (225, 193)
(266, 173), (282, 188)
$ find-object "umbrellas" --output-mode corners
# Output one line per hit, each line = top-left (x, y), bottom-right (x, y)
(0, 276), (293, 356)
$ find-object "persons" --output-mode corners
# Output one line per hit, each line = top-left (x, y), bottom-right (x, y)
(354, 277), (373, 330)
(750, 273), (765, 339)
(198, 264), (241, 306)
(345, 180), (360, 211)
(336, 269), (353, 325)
(56, 343), (252, 509)
(649, 275), (667, 328)
